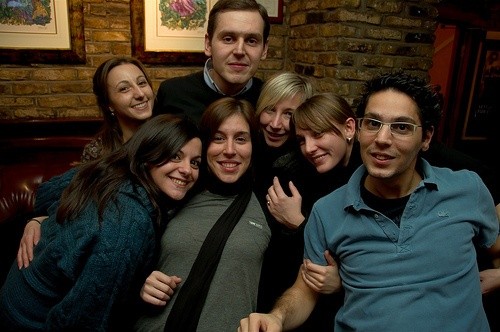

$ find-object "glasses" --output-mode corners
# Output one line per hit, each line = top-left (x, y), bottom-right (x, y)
(356, 118), (422, 137)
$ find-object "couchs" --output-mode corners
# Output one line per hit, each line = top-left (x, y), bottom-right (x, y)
(0, 136), (96, 285)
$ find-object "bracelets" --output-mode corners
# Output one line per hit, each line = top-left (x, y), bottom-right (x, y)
(26, 219), (42, 224)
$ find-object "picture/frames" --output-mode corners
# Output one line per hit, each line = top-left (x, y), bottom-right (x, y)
(130, 0), (218, 67)
(256, 0), (284, 25)
(0, 0), (87, 65)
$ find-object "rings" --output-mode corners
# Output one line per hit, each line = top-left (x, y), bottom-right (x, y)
(267, 200), (270, 205)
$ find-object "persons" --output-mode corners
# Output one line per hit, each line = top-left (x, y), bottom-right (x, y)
(151, 0), (271, 123)
(236, 69), (500, 332)
(0, 113), (208, 332)
(266, 92), (364, 246)
(253, 71), (346, 243)
(16, 56), (159, 269)
(136, 96), (344, 332)
(473, 201), (500, 295)
